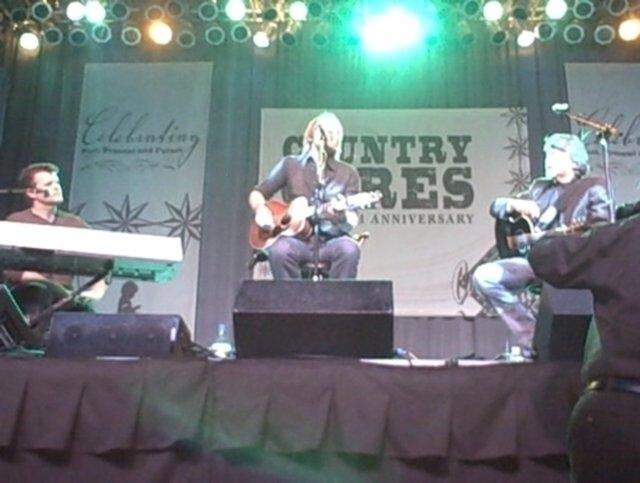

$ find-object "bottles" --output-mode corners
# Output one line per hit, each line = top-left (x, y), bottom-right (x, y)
(208, 322), (234, 359)
(512, 346), (522, 362)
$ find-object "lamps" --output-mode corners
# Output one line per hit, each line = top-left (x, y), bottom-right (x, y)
(545, 0), (568, 20)
(516, 30), (535, 47)
(86, 2), (105, 23)
(288, 1), (309, 21)
(150, 21), (172, 45)
(253, 32), (270, 48)
(19, 20), (41, 51)
(66, 0), (85, 21)
(483, 0), (504, 22)
(618, 19), (640, 42)
(225, 0), (248, 22)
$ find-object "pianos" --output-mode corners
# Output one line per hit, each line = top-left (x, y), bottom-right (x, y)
(1, 222), (184, 283)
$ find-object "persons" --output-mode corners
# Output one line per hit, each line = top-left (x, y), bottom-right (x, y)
(526, 201), (639, 483)
(248, 111), (363, 280)
(470, 130), (612, 358)
(1, 161), (95, 290)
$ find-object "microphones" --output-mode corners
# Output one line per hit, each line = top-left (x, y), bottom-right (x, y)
(319, 124), (327, 140)
(551, 103), (570, 114)
(42, 188), (53, 199)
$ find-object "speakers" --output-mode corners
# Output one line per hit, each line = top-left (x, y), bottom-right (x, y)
(233, 280), (393, 359)
(45, 311), (191, 360)
(532, 282), (594, 364)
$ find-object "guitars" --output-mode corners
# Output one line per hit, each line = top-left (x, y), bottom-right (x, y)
(495, 214), (589, 258)
(249, 189), (383, 250)
